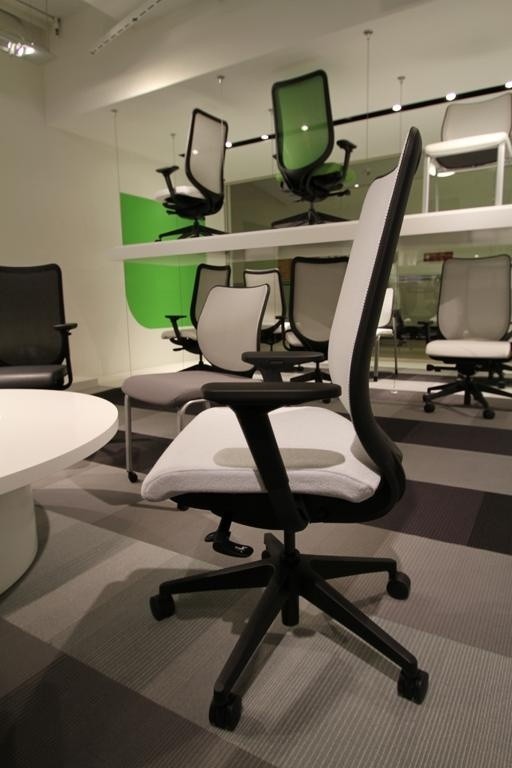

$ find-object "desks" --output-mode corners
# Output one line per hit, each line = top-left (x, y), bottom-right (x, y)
(0, 388), (120, 598)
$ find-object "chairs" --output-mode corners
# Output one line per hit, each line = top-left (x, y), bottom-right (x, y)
(421, 86), (512, 214)
(153, 106), (228, 242)
(121, 283), (271, 483)
(0, 262), (78, 390)
(373, 289), (400, 382)
(270, 69), (358, 228)
(161, 261), (232, 373)
(422, 253), (511, 419)
(242, 268), (299, 354)
(281, 255), (350, 405)
(139, 127), (428, 732)
